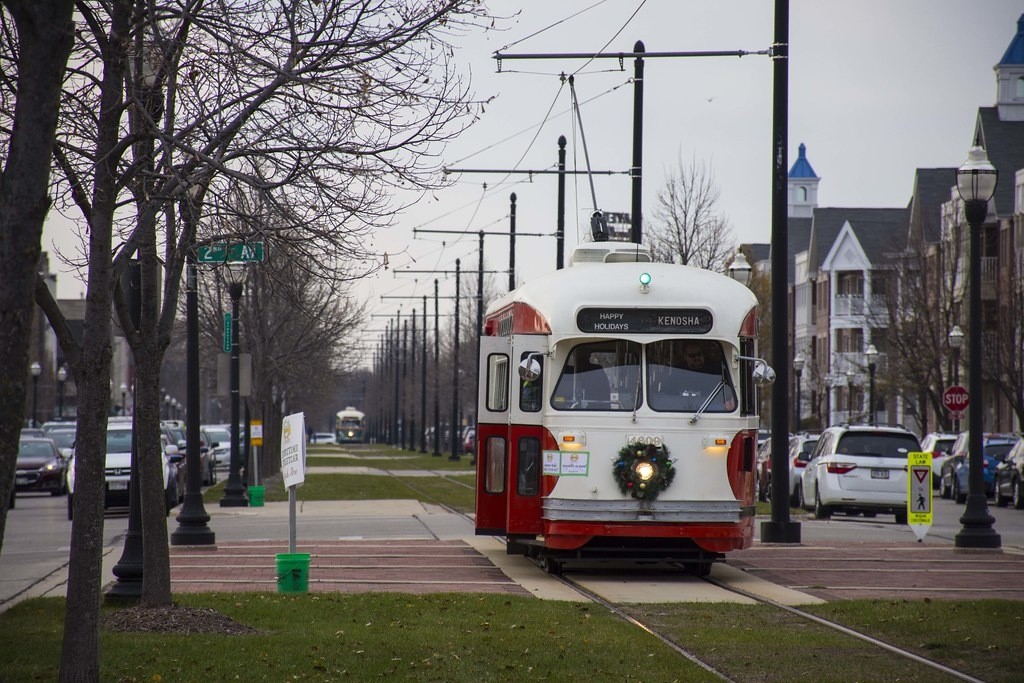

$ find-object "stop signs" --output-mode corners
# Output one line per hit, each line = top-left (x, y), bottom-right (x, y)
(943, 385), (969, 413)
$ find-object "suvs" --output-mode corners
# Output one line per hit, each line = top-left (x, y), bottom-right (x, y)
(756, 422), (924, 525)
(918, 430), (1024, 509)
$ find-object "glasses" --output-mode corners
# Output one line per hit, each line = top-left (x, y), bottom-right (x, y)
(686, 350), (704, 358)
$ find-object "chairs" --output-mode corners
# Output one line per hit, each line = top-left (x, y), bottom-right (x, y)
(576, 364), (610, 409)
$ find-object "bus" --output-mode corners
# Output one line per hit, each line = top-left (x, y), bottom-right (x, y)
(334, 405), (367, 443)
(474, 71), (775, 575)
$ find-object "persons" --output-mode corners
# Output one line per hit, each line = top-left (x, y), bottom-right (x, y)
(307, 426), (316, 443)
(522, 346), (610, 412)
(667, 344), (715, 396)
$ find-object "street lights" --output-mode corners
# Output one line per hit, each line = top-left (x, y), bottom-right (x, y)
(171, 183), (217, 546)
(57, 367), (67, 417)
(31, 361), (41, 428)
(120, 383), (128, 416)
(218, 261), (252, 508)
(956, 144), (1003, 548)
(164, 394), (182, 420)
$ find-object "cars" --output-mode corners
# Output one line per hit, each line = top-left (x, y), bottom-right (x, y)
(16, 416), (245, 520)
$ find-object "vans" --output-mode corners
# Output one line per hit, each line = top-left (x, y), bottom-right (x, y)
(310, 432), (338, 445)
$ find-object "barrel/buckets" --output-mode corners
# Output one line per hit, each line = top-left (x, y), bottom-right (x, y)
(247, 485), (265, 507)
(274, 553), (311, 595)
